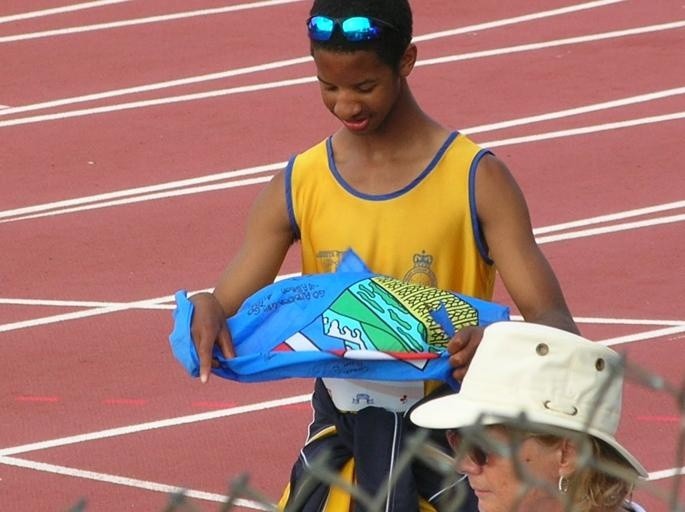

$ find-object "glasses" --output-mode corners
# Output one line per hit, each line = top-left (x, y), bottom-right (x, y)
(446, 430), (487, 466)
(305, 15), (402, 44)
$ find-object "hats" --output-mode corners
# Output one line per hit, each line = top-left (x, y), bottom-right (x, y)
(405, 319), (649, 481)
